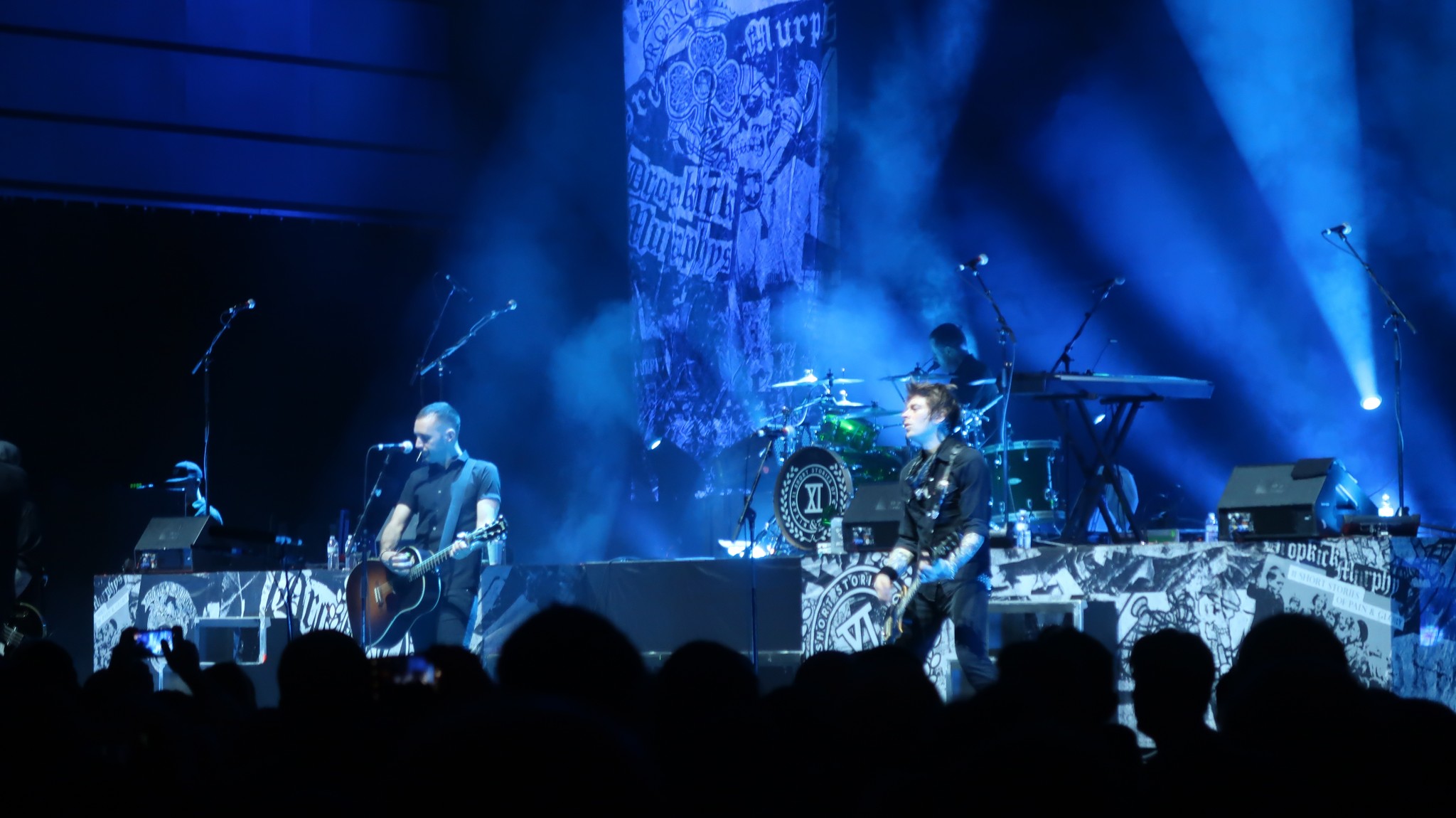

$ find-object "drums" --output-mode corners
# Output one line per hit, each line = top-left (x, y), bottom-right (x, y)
(770, 442), (909, 553)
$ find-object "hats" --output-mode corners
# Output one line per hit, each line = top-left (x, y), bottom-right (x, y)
(167, 460), (204, 490)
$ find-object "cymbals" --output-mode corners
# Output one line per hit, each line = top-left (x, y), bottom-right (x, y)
(835, 405), (903, 422)
(835, 400), (871, 409)
(877, 370), (962, 383)
(771, 373), (819, 389)
(796, 376), (865, 387)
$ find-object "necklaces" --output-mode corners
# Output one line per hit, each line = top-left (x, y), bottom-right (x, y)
(907, 447), (939, 500)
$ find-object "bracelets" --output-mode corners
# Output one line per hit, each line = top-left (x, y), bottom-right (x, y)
(877, 567), (898, 583)
(945, 557), (957, 581)
(379, 549), (389, 563)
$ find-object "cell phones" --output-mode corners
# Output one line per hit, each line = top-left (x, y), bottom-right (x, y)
(131, 629), (174, 658)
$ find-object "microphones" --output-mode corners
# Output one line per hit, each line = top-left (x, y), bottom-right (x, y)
(1325, 224), (1351, 238)
(958, 253), (989, 275)
(374, 439), (412, 454)
(1084, 276), (1128, 295)
(226, 299), (255, 316)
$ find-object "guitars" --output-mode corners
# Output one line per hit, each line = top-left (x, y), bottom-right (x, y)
(345, 513), (511, 651)
(880, 530), (963, 647)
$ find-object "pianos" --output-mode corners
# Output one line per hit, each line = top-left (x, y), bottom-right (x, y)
(996, 362), (1216, 402)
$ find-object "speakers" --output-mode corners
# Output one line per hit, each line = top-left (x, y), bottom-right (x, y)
(133, 516), (221, 571)
(1219, 455), (1378, 543)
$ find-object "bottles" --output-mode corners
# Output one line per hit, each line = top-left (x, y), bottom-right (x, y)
(345, 535), (357, 569)
(1379, 494), (1393, 516)
(1206, 511), (1220, 541)
(1014, 511), (1029, 532)
(327, 535), (341, 572)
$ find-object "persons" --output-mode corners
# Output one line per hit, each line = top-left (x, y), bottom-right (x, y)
(166, 459), (224, 542)
(0, 609), (1456, 818)
(873, 376), (1001, 691)
(930, 322), (1005, 465)
(0, 438), (58, 610)
(378, 401), (502, 659)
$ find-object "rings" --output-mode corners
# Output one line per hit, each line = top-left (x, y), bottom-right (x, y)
(455, 552), (459, 556)
